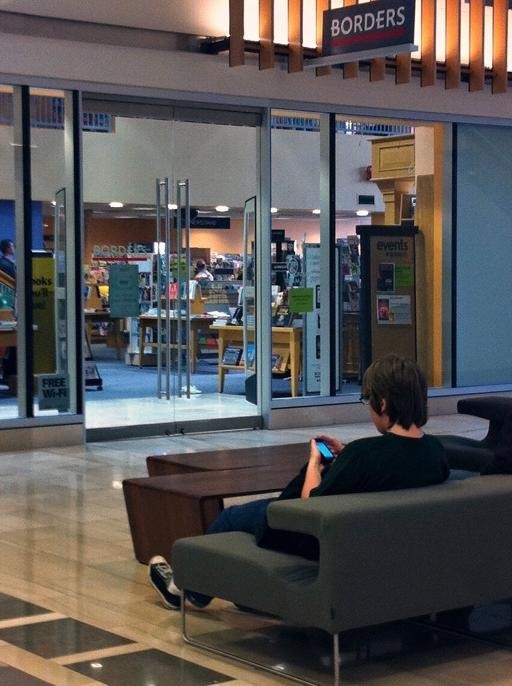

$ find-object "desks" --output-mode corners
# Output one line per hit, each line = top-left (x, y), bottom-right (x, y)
(84, 313), (125, 361)
(207, 324), (303, 397)
(136, 315), (216, 373)
(121, 465), (349, 567)
(146, 442), (310, 531)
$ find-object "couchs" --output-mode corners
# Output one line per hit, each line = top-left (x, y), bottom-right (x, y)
(166, 474), (512, 686)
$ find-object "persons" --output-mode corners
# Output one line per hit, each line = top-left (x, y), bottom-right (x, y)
(194, 259), (215, 282)
(0, 240), (16, 279)
(147, 353), (450, 611)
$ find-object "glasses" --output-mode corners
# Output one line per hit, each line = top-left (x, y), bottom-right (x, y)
(359, 397), (369, 405)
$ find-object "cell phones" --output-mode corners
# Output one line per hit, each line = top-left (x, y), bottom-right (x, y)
(310, 437), (335, 460)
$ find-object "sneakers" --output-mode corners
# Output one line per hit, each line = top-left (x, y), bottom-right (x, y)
(185, 385), (201, 393)
(185, 590), (212, 608)
(148, 555), (181, 609)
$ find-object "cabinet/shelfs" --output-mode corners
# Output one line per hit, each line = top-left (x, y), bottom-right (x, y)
(382, 189), (416, 226)
